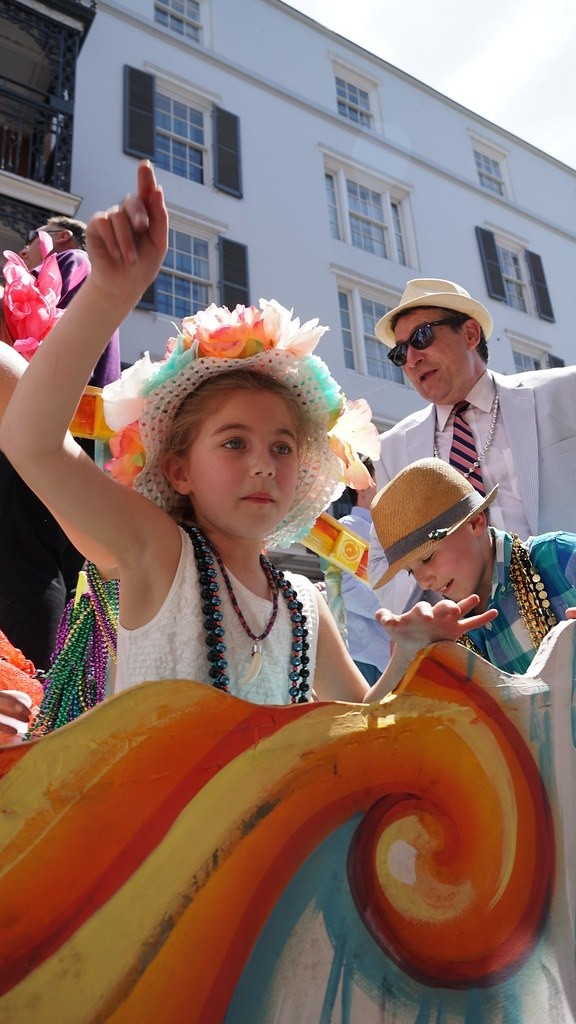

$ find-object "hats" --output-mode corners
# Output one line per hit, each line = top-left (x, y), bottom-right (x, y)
(369, 457), (499, 589)
(102, 298), (380, 553)
(375, 278), (493, 352)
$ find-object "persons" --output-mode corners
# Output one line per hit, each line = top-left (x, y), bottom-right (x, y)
(0, 217), (121, 676)
(322, 456), (394, 688)
(366, 278), (575, 613)
(370, 458), (576, 674)
(0, 160), (498, 714)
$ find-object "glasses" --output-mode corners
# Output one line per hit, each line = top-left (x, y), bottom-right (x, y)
(25, 230), (63, 245)
(387, 320), (462, 366)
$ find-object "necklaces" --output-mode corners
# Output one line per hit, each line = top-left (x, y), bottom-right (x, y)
(23, 561), (121, 741)
(433, 393), (499, 479)
(457, 535), (557, 662)
(181, 524), (310, 704)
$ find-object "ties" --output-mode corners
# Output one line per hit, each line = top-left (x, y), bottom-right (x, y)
(449, 401), (486, 497)
